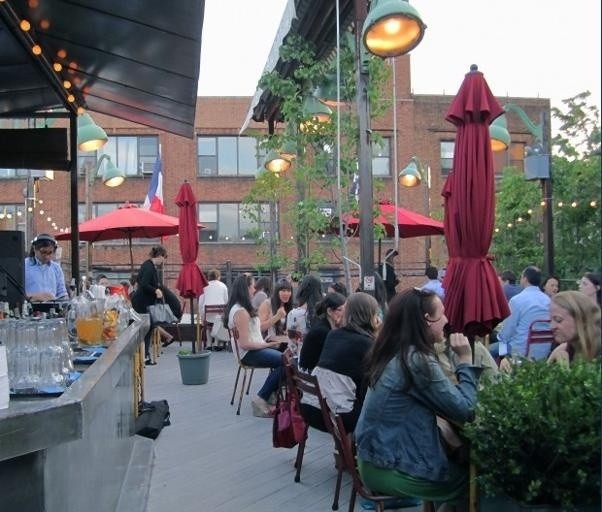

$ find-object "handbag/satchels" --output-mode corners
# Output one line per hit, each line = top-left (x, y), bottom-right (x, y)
(150, 296), (172, 324)
(273, 386), (308, 448)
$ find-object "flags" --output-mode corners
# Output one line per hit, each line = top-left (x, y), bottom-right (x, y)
(139, 153), (164, 218)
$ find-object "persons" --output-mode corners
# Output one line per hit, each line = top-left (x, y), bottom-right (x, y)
(198, 270), (229, 352)
(80, 274), (133, 297)
(489, 265), (601, 375)
(422, 266), (445, 297)
(353, 285), (480, 512)
(380, 248), (400, 298)
(130, 245), (185, 365)
(222, 272), (382, 453)
(22, 232), (69, 317)
(158, 287), (181, 347)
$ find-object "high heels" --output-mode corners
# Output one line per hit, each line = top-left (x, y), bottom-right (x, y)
(251, 400), (272, 417)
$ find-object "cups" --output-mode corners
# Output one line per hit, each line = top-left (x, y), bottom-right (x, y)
(1, 317), (80, 395)
(67, 297), (105, 346)
(101, 309), (119, 340)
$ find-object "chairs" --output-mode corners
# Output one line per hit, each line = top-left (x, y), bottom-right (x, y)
(526, 318), (556, 360)
(228, 321), (289, 417)
(284, 363), (353, 488)
(321, 395), (436, 511)
(157, 300), (188, 345)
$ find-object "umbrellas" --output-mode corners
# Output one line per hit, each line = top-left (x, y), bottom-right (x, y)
(174, 178), (207, 355)
(52, 202), (207, 290)
(444, 62), (511, 512)
(305, 197), (444, 265)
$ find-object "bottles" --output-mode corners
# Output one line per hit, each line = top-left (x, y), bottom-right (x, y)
(105, 284), (131, 329)
(1, 299), (64, 320)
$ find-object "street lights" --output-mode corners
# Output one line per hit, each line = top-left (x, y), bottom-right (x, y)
(84, 152), (127, 276)
(398, 154), (432, 272)
(23, 102), (108, 257)
(353, 0), (427, 297)
(293, 85), (334, 278)
(487, 100), (556, 278)
(264, 148), (292, 288)
